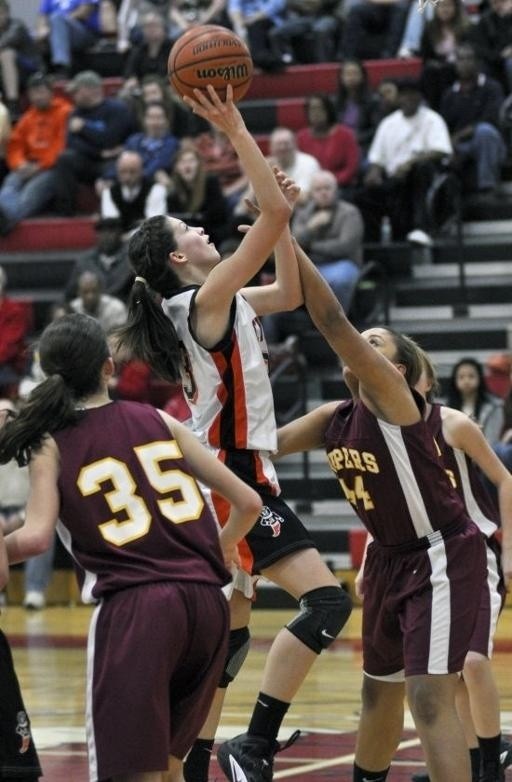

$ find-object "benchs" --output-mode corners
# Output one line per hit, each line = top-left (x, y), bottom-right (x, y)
(274, 219), (475, 568)
(196, 58), (422, 189)
(0, 74), (125, 411)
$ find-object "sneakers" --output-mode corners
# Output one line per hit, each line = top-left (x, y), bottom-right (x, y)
(483, 740), (512, 778)
(1, 590), (46, 610)
(218, 736), (276, 781)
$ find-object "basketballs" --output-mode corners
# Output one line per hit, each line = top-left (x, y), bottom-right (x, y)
(168, 23), (253, 104)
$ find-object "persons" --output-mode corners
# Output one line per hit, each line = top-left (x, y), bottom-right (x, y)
(3, 311), (262, 781)
(0, 399), (55, 609)
(1, 516), (44, 782)
(113, 82), (354, 781)
(236, 197), (488, 782)
(443, 357), (505, 446)
(408, 340), (512, 781)
